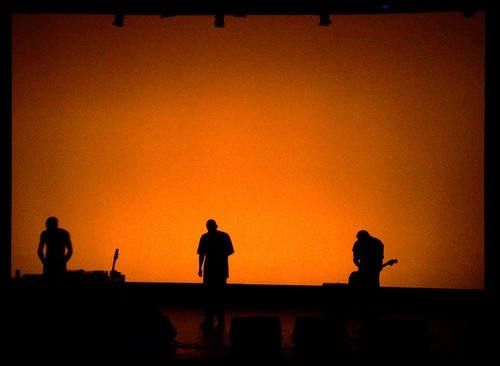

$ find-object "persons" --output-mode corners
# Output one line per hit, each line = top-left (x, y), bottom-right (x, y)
(197, 219), (235, 329)
(38, 216), (73, 274)
(352, 230), (384, 323)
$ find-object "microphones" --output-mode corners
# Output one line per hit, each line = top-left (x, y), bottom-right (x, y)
(113, 248), (119, 260)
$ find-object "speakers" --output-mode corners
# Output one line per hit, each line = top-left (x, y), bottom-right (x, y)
(354, 318), (430, 349)
(227, 316), (283, 348)
(290, 317), (350, 348)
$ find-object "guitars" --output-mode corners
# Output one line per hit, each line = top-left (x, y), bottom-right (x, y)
(347, 258), (398, 287)
(108, 248), (119, 278)
